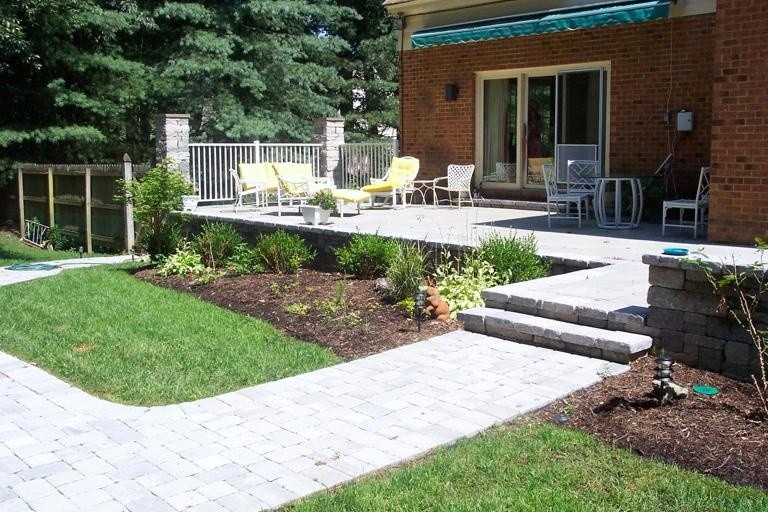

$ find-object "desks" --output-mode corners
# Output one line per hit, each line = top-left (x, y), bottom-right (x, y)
(573, 176), (660, 230)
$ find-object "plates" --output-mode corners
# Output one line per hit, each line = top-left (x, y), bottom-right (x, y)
(662, 246), (689, 257)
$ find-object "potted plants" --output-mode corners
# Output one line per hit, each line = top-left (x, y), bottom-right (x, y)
(180, 181), (200, 213)
(300, 188), (337, 227)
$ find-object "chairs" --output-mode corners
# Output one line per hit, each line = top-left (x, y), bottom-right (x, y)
(228, 156), (476, 218)
(542, 163), (590, 229)
(662, 166), (710, 240)
